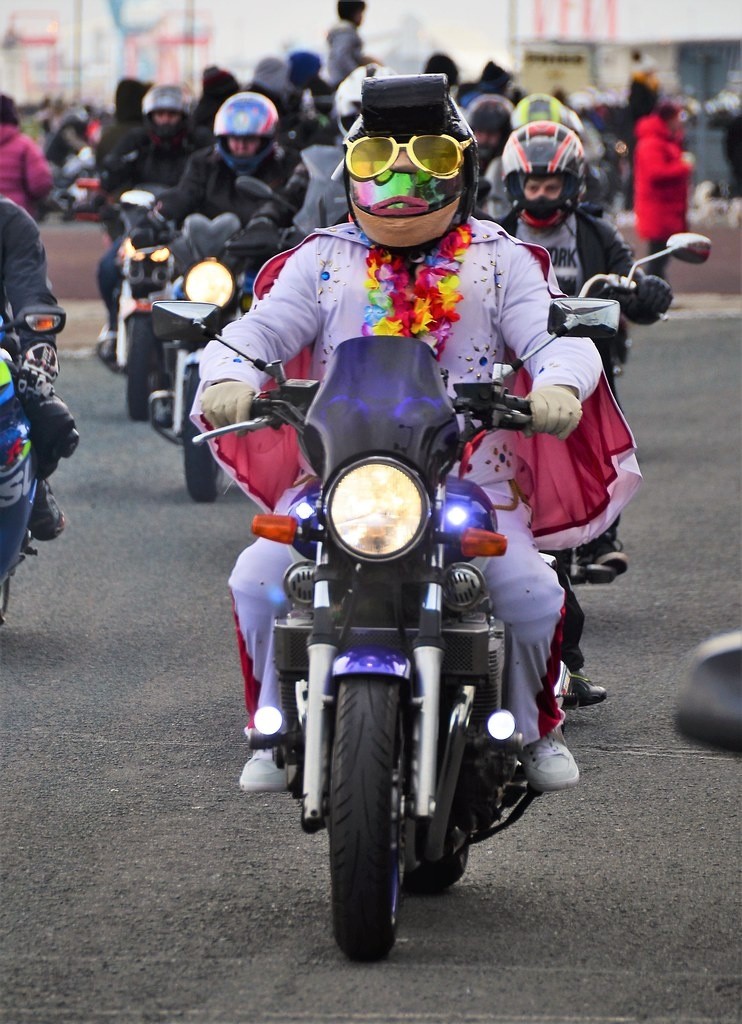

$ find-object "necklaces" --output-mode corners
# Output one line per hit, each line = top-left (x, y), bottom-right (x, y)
(362, 223), (472, 360)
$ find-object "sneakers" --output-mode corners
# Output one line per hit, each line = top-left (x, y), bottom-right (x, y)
(26, 478), (67, 542)
(588, 538), (629, 576)
(516, 734), (579, 792)
(562, 669), (607, 708)
(239, 746), (289, 792)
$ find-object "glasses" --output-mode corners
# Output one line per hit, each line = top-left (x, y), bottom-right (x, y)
(344, 132), (474, 180)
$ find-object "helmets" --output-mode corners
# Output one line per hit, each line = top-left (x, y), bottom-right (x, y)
(213, 92), (280, 176)
(501, 120), (589, 229)
(464, 93), (515, 170)
(332, 66), (394, 142)
(336, 75), (482, 249)
(507, 84), (630, 163)
(140, 78), (197, 150)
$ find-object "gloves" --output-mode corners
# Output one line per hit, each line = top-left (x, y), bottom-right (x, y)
(199, 380), (257, 437)
(16, 335), (61, 406)
(241, 216), (278, 256)
(635, 274), (673, 315)
(521, 384), (583, 442)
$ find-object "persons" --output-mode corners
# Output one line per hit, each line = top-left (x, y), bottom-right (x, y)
(557, 569), (607, 707)
(0, 197), (77, 540)
(617, 52), (656, 226)
(195, 74), (641, 799)
(325, 0), (380, 81)
(423, 54), (618, 210)
(40, 52), (394, 362)
(0, 95), (53, 219)
(633, 104), (694, 276)
(708, 93), (742, 224)
(494, 121), (673, 574)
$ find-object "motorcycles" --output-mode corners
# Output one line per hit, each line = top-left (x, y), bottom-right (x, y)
(49, 144), (102, 222)
(97, 145), (349, 502)
(0, 306), (67, 623)
(151, 231), (712, 961)
